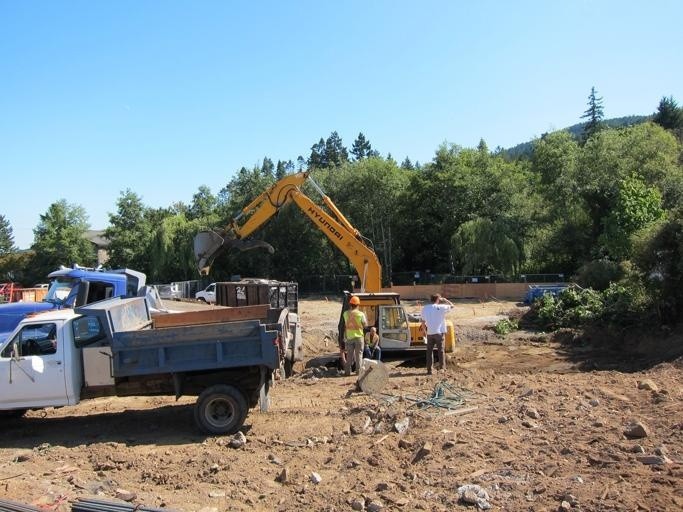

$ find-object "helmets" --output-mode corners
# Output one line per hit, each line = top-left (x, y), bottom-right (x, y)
(349, 296), (361, 305)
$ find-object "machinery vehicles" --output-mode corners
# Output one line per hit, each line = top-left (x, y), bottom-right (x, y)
(188, 171), (456, 362)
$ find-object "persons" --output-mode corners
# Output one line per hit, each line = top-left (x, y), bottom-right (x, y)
(420, 294), (454, 374)
(341, 296), (381, 375)
